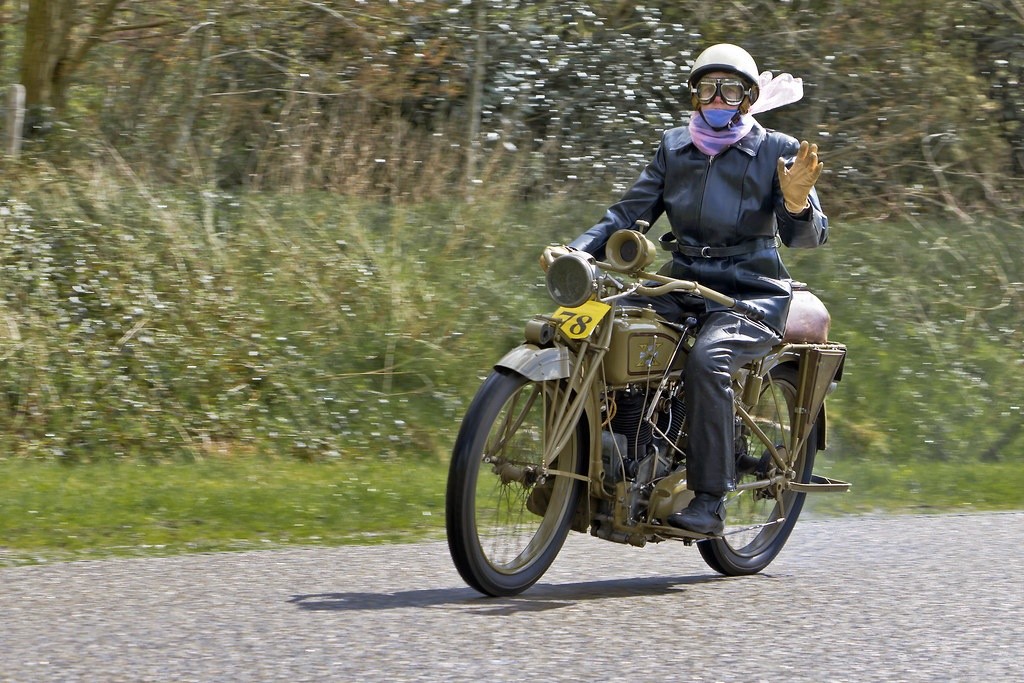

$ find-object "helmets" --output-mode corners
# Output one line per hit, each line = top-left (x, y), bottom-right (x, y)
(688, 42), (760, 88)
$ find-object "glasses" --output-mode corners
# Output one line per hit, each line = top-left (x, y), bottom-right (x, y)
(691, 77), (752, 107)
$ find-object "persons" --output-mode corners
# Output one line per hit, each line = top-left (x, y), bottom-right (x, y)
(539, 44), (828, 536)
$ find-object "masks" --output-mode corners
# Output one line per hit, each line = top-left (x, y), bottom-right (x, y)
(700, 108), (738, 128)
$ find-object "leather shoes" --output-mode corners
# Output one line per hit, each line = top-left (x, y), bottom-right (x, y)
(666, 490), (728, 536)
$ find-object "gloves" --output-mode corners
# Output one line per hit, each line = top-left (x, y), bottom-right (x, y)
(777, 139), (824, 215)
(538, 242), (576, 274)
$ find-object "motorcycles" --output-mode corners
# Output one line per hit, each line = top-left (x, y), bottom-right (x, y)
(444, 219), (853, 598)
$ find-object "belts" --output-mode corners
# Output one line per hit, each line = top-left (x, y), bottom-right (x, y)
(657, 231), (782, 260)
